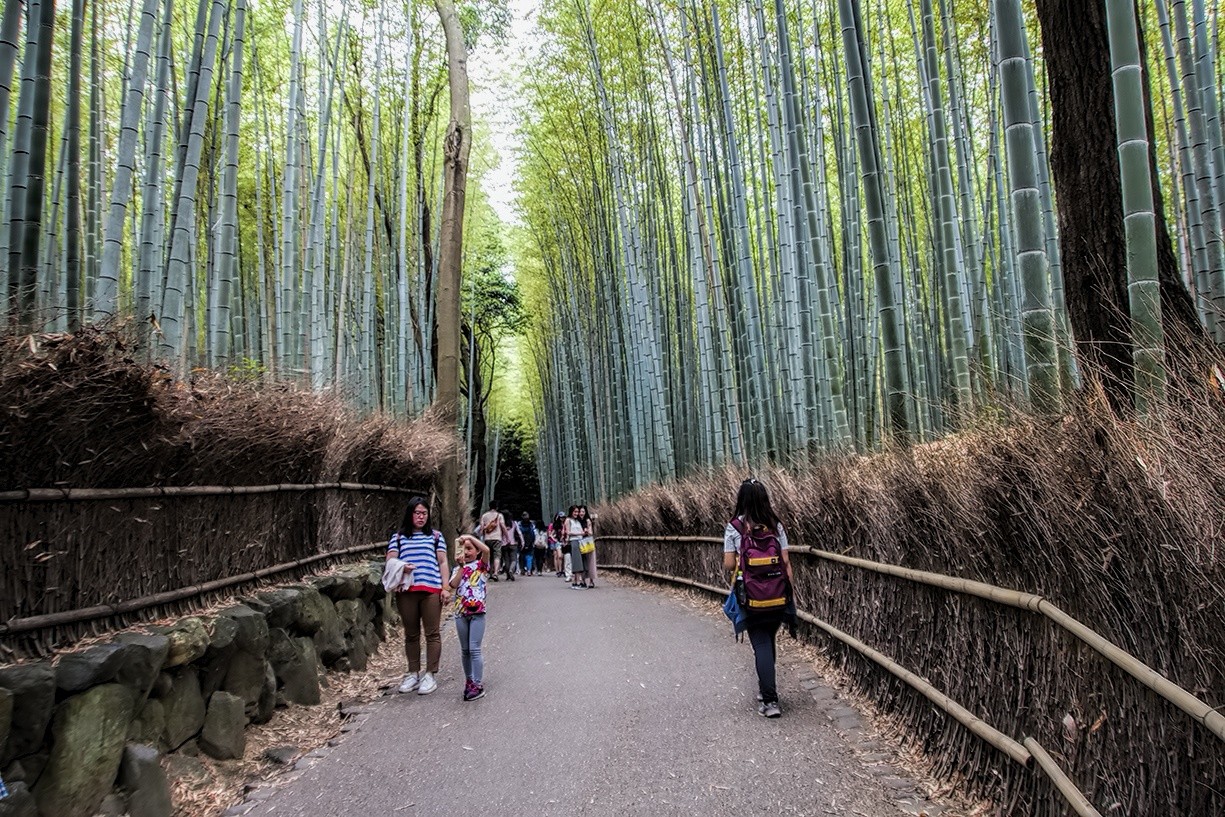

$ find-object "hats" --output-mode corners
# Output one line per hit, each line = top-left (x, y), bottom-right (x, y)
(558, 512), (566, 517)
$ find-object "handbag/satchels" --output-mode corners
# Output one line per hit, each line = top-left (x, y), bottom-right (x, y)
(578, 533), (595, 554)
(483, 514), (499, 534)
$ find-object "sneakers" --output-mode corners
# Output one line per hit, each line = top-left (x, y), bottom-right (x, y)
(464, 679), (474, 695)
(757, 701), (781, 717)
(757, 694), (763, 700)
(464, 684), (485, 701)
(398, 675), (421, 693)
(418, 675), (438, 694)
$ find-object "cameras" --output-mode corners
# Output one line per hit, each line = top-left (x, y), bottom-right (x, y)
(454, 542), (465, 561)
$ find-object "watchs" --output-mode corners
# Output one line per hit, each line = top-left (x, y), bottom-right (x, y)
(442, 585), (451, 592)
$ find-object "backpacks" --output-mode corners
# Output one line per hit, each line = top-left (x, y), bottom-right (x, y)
(730, 518), (787, 613)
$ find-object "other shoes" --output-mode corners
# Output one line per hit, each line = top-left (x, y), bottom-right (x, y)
(565, 578), (571, 582)
(527, 571), (531, 576)
(492, 576), (498, 581)
(508, 571), (516, 581)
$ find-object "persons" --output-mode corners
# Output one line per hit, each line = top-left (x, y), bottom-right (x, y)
(477, 501), (598, 590)
(724, 479), (794, 717)
(448, 535), (490, 700)
(386, 496), (452, 696)
